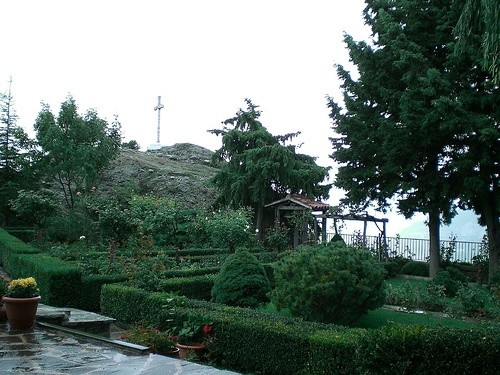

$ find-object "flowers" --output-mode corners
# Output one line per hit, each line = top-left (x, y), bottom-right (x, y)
(0, 275), (12, 306)
(119, 326), (178, 352)
(6, 276), (40, 298)
(173, 320), (213, 346)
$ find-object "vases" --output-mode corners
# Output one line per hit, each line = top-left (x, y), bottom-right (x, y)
(159, 346), (180, 358)
(0, 298), (9, 325)
(146, 344), (154, 354)
(1, 292), (42, 330)
(176, 341), (206, 359)
